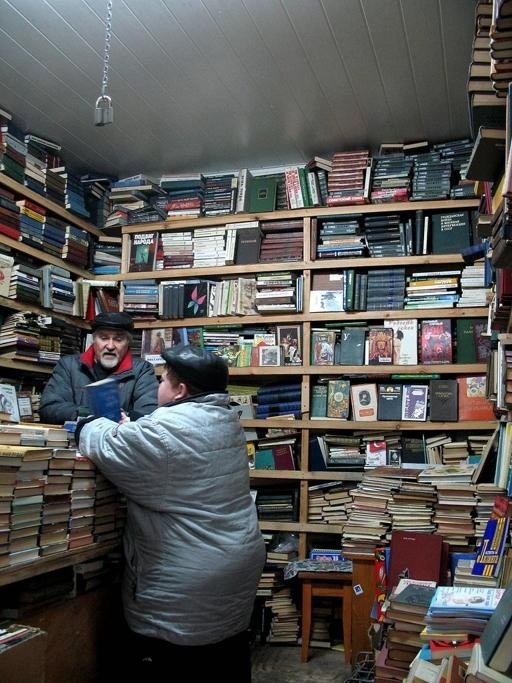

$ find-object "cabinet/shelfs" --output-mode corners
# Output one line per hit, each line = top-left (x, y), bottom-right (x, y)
(1, 161), (512, 682)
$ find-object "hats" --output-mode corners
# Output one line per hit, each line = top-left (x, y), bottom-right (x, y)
(161, 345), (228, 393)
(91, 312), (134, 335)
